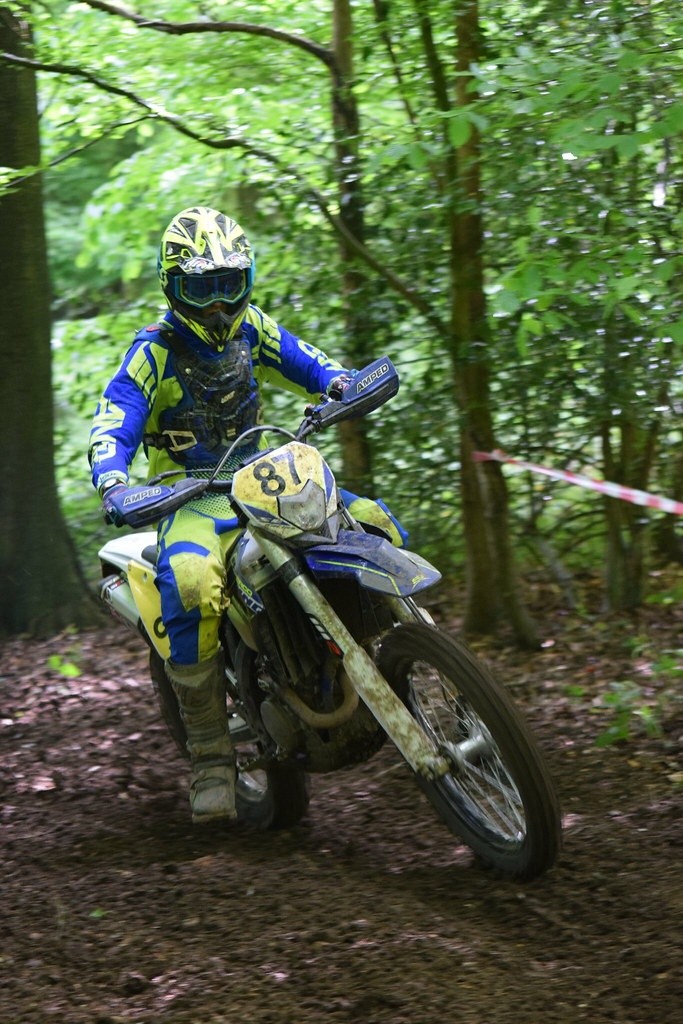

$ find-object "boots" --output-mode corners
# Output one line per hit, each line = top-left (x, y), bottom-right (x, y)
(164, 646), (237, 823)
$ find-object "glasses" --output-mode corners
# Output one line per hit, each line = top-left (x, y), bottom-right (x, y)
(174, 269), (253, 309)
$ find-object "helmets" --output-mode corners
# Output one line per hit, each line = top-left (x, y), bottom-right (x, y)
(157, 207), (255, 352)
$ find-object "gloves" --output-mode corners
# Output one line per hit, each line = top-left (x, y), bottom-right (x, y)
(103, 484), (129, 528)
(329, 369), (359, 401)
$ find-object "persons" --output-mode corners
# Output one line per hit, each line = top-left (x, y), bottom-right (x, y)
(88, 206), (409, 824)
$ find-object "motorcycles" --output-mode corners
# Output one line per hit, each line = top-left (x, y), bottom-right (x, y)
(96, 356), (561, 883)
